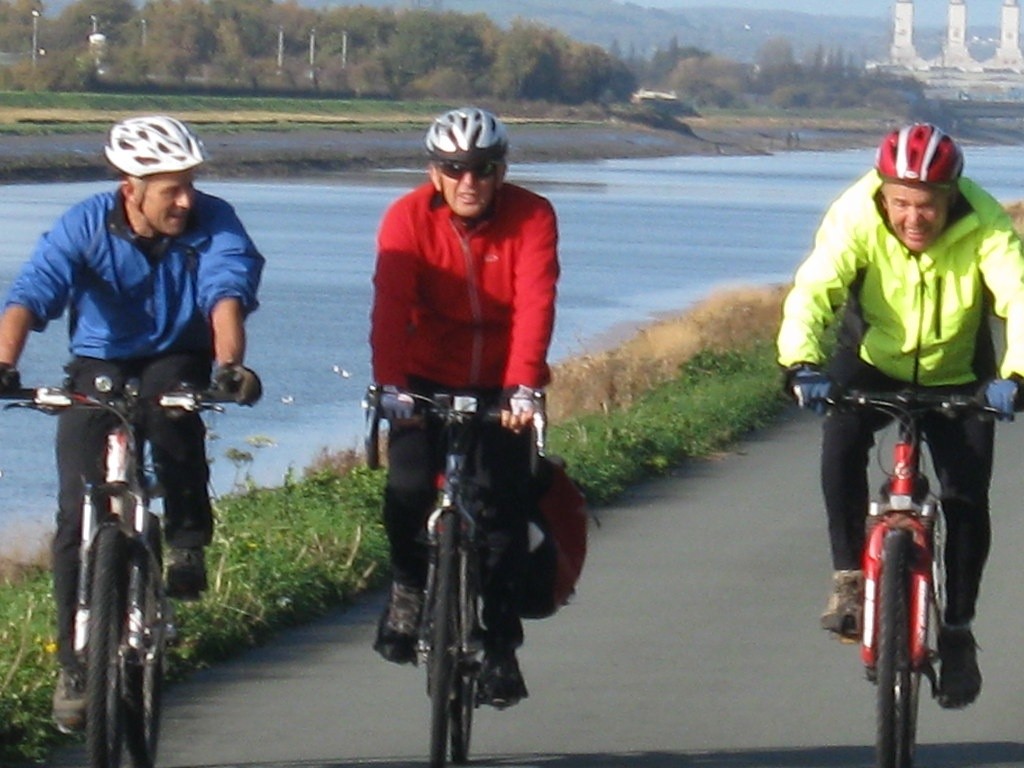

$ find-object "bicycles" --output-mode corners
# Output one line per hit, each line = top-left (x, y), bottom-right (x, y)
(795, 381), (1015, 768)
(362, 381), (548, 768)
(0, 381), (250, 768)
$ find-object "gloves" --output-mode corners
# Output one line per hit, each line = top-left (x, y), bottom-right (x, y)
(788, 368), (839, 415)
(214, 362), (262, 408)
(985, 379), (1019, 423)
(0, 362), (22, 398)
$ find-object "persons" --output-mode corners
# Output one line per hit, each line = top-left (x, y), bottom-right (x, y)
(370, 108), (560, 708)
(0, 114), (264, 734)
(778, 123), (1024, 707)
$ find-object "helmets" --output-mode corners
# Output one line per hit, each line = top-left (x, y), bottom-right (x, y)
(875, 123), (965, 184)
(103, 116), (205, 178)
(425, 107), (509, 162)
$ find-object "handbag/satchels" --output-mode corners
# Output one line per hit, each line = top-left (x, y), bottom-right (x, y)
(520, 455), (600, 620)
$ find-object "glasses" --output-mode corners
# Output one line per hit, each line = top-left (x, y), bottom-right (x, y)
(434, 161), (499, 181)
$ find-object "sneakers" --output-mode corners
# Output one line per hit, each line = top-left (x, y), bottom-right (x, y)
(485, 652), (519, 706)
(166, 549), (206, 591)
(52, 664), (88, 734)
(820, 571), (863, 630)
(377, 581), (421, 662)
(938, 630), (983, 707)
